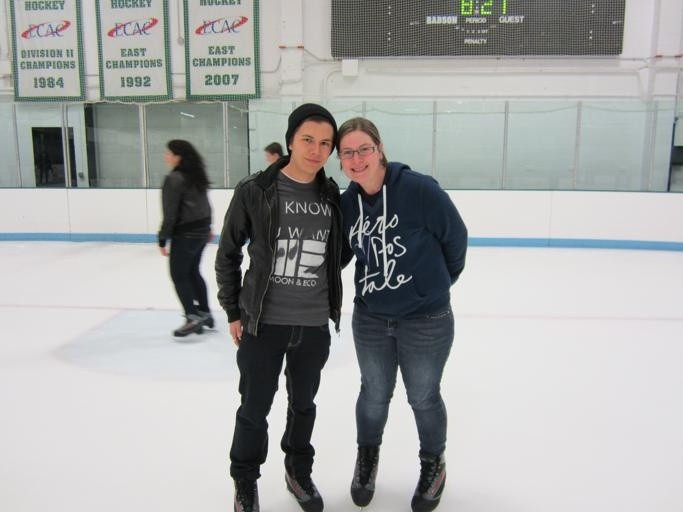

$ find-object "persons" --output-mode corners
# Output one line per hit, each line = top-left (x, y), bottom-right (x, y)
(35, 144), (54, 186)
(263, 141), (283, 165)
(155, 138), (215, 338)
(214, 101), (347, 512)
(332, 115), (468, 512)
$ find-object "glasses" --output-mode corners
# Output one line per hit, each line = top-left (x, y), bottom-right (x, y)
(339, 146), (378, 160)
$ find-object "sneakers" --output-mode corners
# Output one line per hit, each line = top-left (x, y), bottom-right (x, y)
(232, 474), (261, 512)
(410, 448), (448, 511)
(197, 310), (214, 328)
(174, 312), (204, 336)
(349, 444), (380, 507)
(283, 453), (324, 512)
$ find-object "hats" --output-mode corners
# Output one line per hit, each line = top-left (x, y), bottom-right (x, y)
(285, 102), (337, 156)
